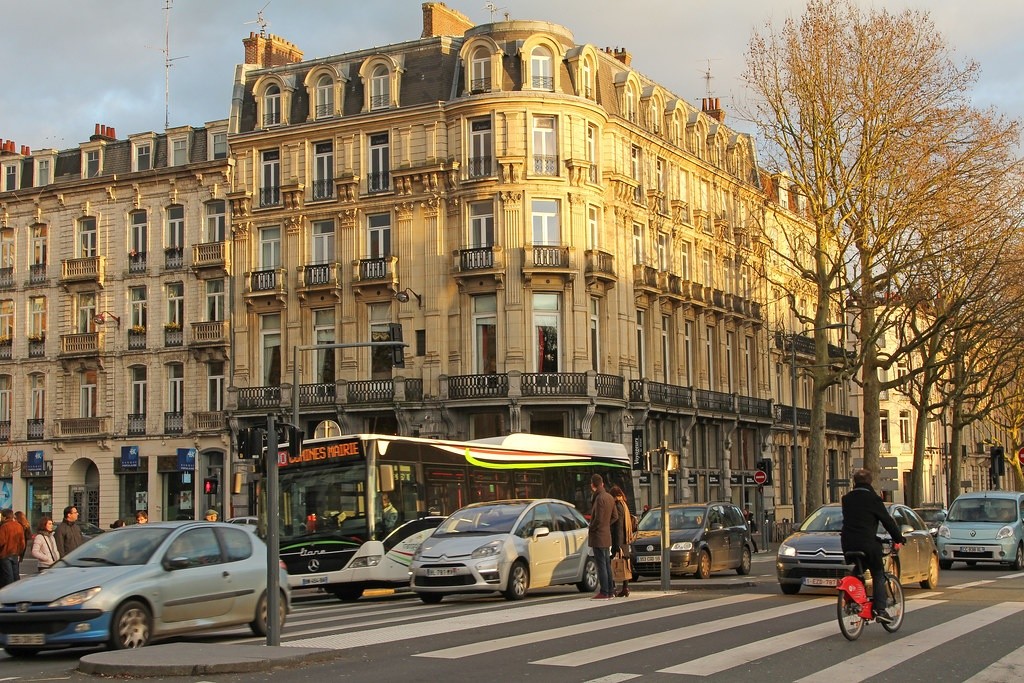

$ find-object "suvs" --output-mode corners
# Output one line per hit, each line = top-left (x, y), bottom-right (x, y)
(630, 500), (757, 578)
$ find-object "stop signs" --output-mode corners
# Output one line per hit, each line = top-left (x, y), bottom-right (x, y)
(754, 471), (769, 485)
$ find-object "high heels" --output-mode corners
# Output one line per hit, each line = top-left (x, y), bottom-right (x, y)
(613, 587), (630, 598)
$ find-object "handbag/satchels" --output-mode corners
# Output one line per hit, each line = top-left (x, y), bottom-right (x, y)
(630, 514), (638, 532)
(610, 546), (632, 582)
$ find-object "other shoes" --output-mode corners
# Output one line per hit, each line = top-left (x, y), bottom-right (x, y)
(876, 610), (895, 623)
(590, 592), (613, 600)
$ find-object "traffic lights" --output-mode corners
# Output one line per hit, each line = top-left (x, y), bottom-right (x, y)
(204, 478), (218, 495)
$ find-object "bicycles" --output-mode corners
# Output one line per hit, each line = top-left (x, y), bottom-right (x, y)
(836, 538), (907, 640)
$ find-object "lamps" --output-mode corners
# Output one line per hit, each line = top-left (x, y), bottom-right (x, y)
(396, 288), (421, 310)
(92, 311), (120, 330)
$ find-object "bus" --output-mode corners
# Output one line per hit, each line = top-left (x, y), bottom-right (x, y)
(252, 431), (639, 604)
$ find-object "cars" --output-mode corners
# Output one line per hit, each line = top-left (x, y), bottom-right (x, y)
(773, 503), (940, 590)
(911, 507), (949, 546)
(0, 519), (293, 656)
(408, 498), (603, 604)
(936, 489), (1024, 570)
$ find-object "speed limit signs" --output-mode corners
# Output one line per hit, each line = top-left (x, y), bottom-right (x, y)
(1018, 447), (1024, 466)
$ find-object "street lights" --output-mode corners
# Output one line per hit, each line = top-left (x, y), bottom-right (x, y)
(237, 411), (309, 644)
(791, 322), (853, 521)
(640, 440), (682, 590)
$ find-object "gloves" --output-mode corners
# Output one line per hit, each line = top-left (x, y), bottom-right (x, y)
(895, 536), (906, 546)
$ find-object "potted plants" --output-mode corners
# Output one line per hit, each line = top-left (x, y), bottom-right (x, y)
(164, 322), (181, 333)
(127, 325), (146, 335)
(0, 334), (12, 347)
(29, 334), (44, 345)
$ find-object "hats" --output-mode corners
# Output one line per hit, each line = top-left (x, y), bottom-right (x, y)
(205, 509), (219, 515)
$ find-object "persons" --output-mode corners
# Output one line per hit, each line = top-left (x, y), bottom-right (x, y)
(202, 510), (219, 522)
(0, 509), (32, 589)
(608, 483), (635, 598)
(109, 519), (126, 528)
(589, 474), (619, 600)
(742, 509), (759, 553)
(840, 468), (906, 623)
(641, 504), (648, 518)
(134, 511), (148, 525)
(55, 506), (87, 558)
(30, 515), (60, 571)
(381, 492), (398, 532)
(669, 515), (704, 527)
